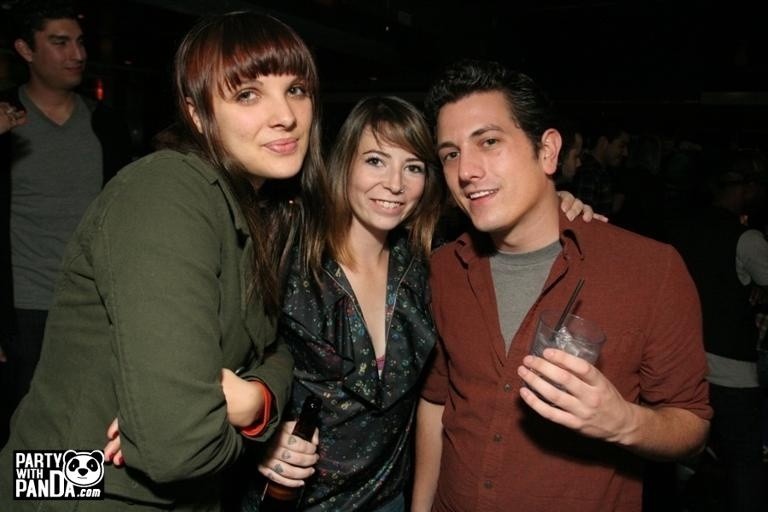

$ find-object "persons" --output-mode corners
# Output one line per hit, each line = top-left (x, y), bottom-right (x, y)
(0, 0), (766, 512)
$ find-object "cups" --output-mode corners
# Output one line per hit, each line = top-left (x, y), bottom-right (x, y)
(527, 308), (607, 410)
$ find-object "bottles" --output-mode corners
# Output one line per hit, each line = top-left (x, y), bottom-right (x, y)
(253, 394), (324, 512)
